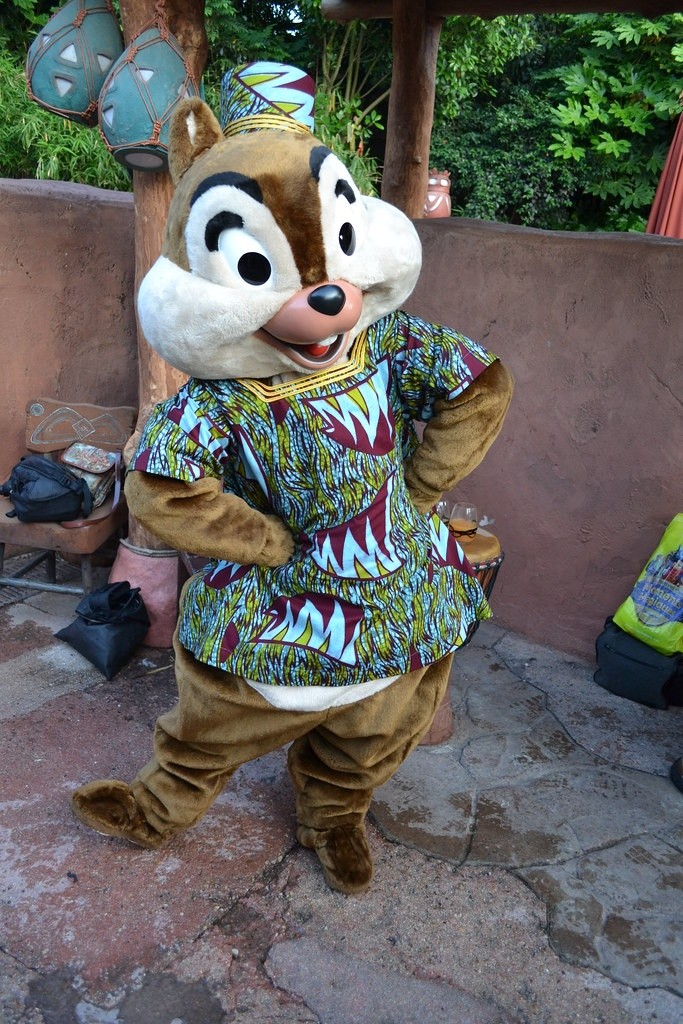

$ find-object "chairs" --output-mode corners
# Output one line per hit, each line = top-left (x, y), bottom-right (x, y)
(1, 396), (137, 599)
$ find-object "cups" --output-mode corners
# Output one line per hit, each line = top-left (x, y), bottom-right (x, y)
(436, 500), (450, 528)
(447, 501), (478, 545)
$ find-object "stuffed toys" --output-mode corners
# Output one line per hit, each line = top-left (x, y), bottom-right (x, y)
(71, 97), (512, 892)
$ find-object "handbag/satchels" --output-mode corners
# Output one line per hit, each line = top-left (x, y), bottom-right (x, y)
(594, 513), (683, 708)
(0, 455), (84, 523)
(53, 580), (151, 680)
(60, 440), (120, 510)
